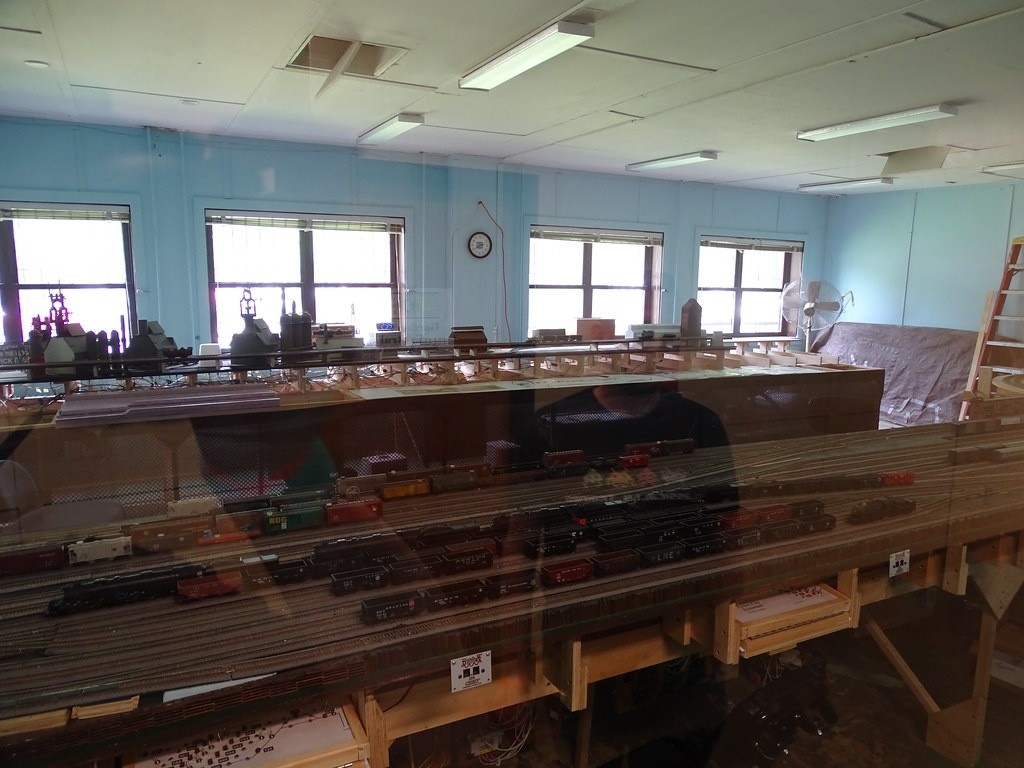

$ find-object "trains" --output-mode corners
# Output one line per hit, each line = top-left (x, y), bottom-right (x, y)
(171, 469), (918, 626)
(331, 436), (701, 501)
(46, 548), (282, 618)
(61, 495), (390, 568)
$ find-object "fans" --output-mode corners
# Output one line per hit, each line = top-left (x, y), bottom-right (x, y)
(782, 279), (843, 353)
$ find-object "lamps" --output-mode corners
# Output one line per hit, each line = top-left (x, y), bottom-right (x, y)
(624, 151), (717, 172)
(797, 177), (893, 193)
(795, 103), (957, 142)
(356, 113), (424, 147)
(979, 162), (1024, 179)
(458, 19), (595, 93)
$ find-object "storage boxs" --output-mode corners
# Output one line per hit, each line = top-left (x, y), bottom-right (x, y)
(375, 330), (401, 348)
(229, 333), (280, 369)
(128, 332), (174, 371)
(576, 318), (616, 340)
(532, 328), (566, 337)
(44, 334), (88, 376)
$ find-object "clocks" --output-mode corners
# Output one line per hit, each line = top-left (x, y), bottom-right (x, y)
(467, 232), (492, 258)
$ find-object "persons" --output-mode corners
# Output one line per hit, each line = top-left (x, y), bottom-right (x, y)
(501, 382), (739, 768)
